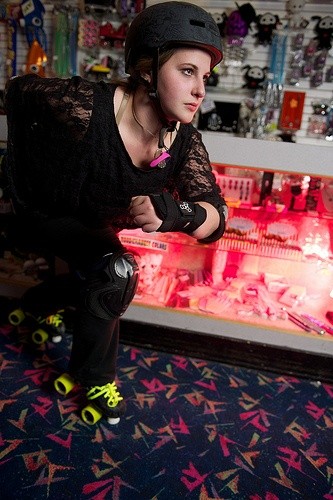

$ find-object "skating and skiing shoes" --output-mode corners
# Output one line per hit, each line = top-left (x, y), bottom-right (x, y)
(10, 292), (67, 344)
(54, 364), (128, 426)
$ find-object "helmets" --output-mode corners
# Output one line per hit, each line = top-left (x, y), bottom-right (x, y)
(124, 0), (224, 77)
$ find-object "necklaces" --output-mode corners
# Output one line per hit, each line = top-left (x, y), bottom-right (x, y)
(132, 96), (169, 139)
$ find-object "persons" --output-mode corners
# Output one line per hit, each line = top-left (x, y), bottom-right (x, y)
(1, 1), (228, 427)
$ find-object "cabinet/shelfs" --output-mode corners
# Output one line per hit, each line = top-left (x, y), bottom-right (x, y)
(115, 132), (333, 359)
